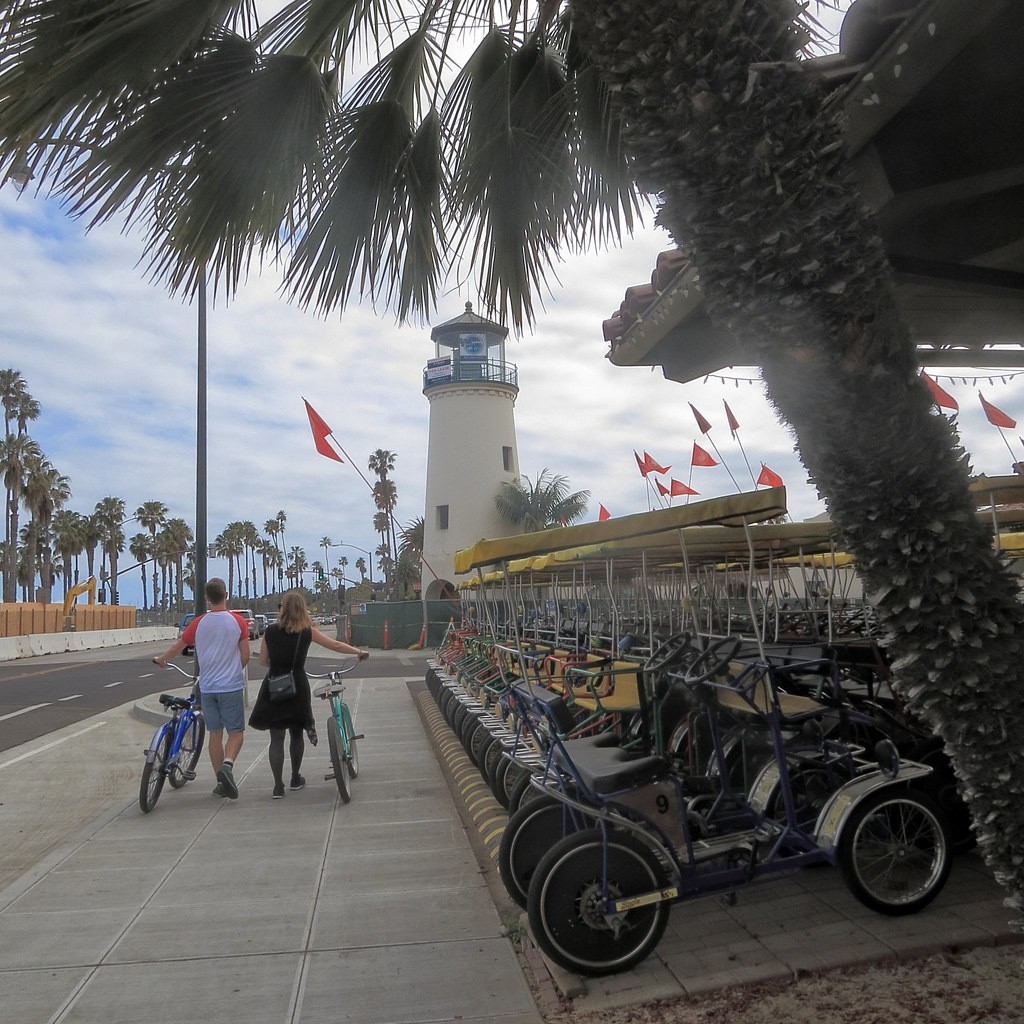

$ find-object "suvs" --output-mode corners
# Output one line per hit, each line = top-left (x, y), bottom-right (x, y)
(230, 608), (260, 640)
(263, 612), (280, 625)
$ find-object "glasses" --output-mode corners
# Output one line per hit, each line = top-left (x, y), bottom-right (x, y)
(278, 603), (283, 609)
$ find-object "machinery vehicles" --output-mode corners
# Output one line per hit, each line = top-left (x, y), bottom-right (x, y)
(62, 575), (98, 632)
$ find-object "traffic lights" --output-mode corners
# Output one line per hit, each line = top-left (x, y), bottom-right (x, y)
(278, 569), (283, 579)
(115, 591), (120, 605)
(319, 568), (323, 580)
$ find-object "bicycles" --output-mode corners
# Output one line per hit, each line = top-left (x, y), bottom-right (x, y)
(305, 654), (366, 803)
(139, 659), (206, 813)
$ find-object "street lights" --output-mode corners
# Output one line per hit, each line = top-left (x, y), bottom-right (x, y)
(331, 544), (372, 582)
(102, 515), (159, 605)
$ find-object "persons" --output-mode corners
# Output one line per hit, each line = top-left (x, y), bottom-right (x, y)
(153, 577), (251, 799)
(248, 592), (369, 799)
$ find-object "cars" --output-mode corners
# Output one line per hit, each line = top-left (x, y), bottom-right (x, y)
(308, 615), (337, 626)
(255, 615), (269, 636)
(174, 613), (196, 656)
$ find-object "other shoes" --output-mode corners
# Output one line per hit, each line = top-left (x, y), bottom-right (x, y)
(217, 766), (240, 800)
(272, 785), (285, 799)
(289, 776), (305, 790)
(213, 784), (227, 797)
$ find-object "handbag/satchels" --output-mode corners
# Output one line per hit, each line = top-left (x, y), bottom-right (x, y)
(267, 675), (296, 700)
